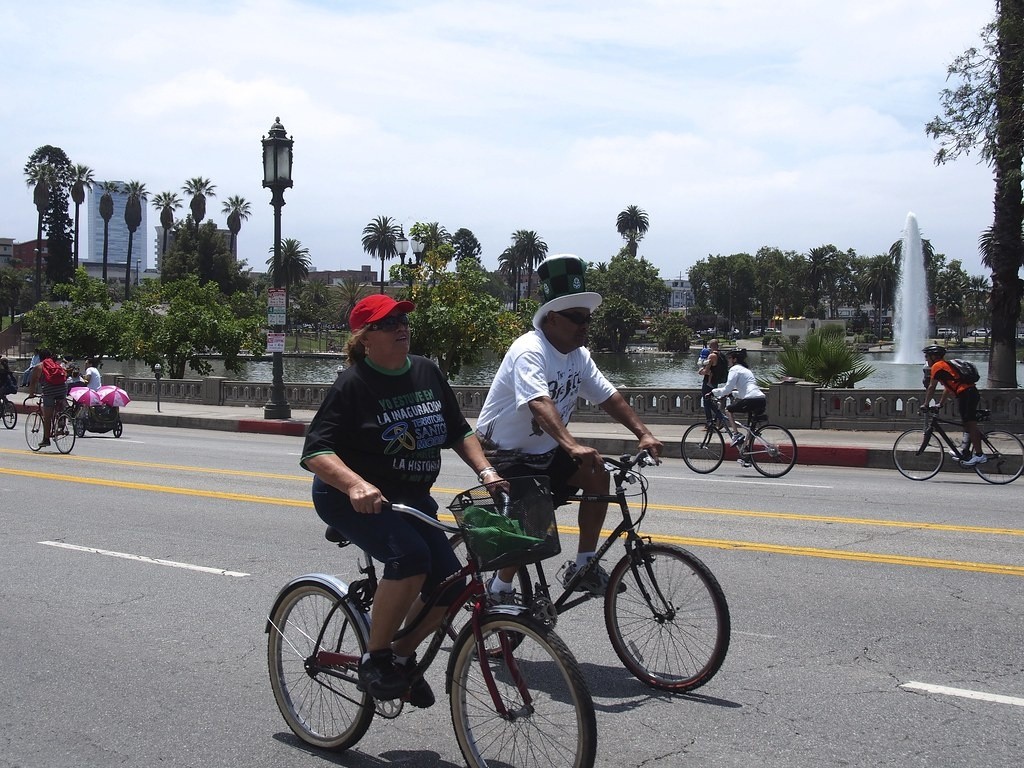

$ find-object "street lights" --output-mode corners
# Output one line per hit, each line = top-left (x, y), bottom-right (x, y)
(394, 224), (426, 302)
(261, 115), (294, 422)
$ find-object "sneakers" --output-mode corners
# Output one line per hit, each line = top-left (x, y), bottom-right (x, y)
(737, 459), (752, 467)
(394, 652), (435, 709)
(965, 454), (987, 465)
(356, 649), (407, 701)
(730, 432), (745, 448)
(562, 560), (626, 595)
(949, 448), (964, 457)
(489, 588), (517, 606)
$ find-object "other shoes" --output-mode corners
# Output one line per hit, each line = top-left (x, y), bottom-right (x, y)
(38, 441), (51, 446)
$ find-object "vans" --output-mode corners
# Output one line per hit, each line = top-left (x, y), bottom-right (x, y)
(938, 328), (957, 337)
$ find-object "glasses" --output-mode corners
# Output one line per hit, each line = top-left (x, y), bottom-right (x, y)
(554, 312), (593, 323)
(364, 314), (408, 331)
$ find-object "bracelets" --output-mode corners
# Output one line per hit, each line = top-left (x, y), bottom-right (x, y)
(478, 466), (498, 482)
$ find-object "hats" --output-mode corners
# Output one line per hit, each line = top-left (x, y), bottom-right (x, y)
(349, 295), (414, 334)
(700, 348), (710, 358)
(532, 255), (602, 330)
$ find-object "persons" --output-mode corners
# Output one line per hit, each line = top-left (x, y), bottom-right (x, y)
(811, 320), (815, 334)
(20, 349), (101, 396)
(698, 339), (728, 431)
(0, 355), (10, 419)
(697, 348), (714, 388)
(711, 348), (767, 467)
(702, 338), (707, 348)
(296, 296), (513, 711)
(28, 349), (68, 446)
(474, 255), (665, 638)
(878, 338), (883, 348)
(919, 346), (986, 466)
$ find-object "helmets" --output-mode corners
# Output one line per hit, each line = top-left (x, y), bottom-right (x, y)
(726, 348), (747, 356)
(922, 345), (946, 354)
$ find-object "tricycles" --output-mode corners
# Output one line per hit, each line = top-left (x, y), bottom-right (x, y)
(65, 381), (123, 438)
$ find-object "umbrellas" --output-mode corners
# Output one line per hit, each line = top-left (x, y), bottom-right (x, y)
(69, 387), (101, 407)
(97, 384), (131, 409)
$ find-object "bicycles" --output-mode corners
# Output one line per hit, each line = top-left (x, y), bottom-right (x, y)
(0, 384), (19, 430)
(892, 404), (1024, 485)
(435, 449), (730, 692)
(265, 478), (598, 768)
(22, 395), (77, 454)
(681, 392), (798, 478)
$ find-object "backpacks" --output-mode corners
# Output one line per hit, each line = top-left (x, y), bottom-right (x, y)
(711, 351), (727, 383)
(0, 369), (18, 394)
(39, 358), (66, 384)
(937, 359), (980, 384)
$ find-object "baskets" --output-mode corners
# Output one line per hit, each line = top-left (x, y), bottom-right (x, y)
(449, 474), (562, 573)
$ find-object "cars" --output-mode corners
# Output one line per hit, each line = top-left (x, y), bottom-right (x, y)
(967, 327), (991, 338)
(695, 326), (781, 339)
(1017, 332), (1024, 337)
